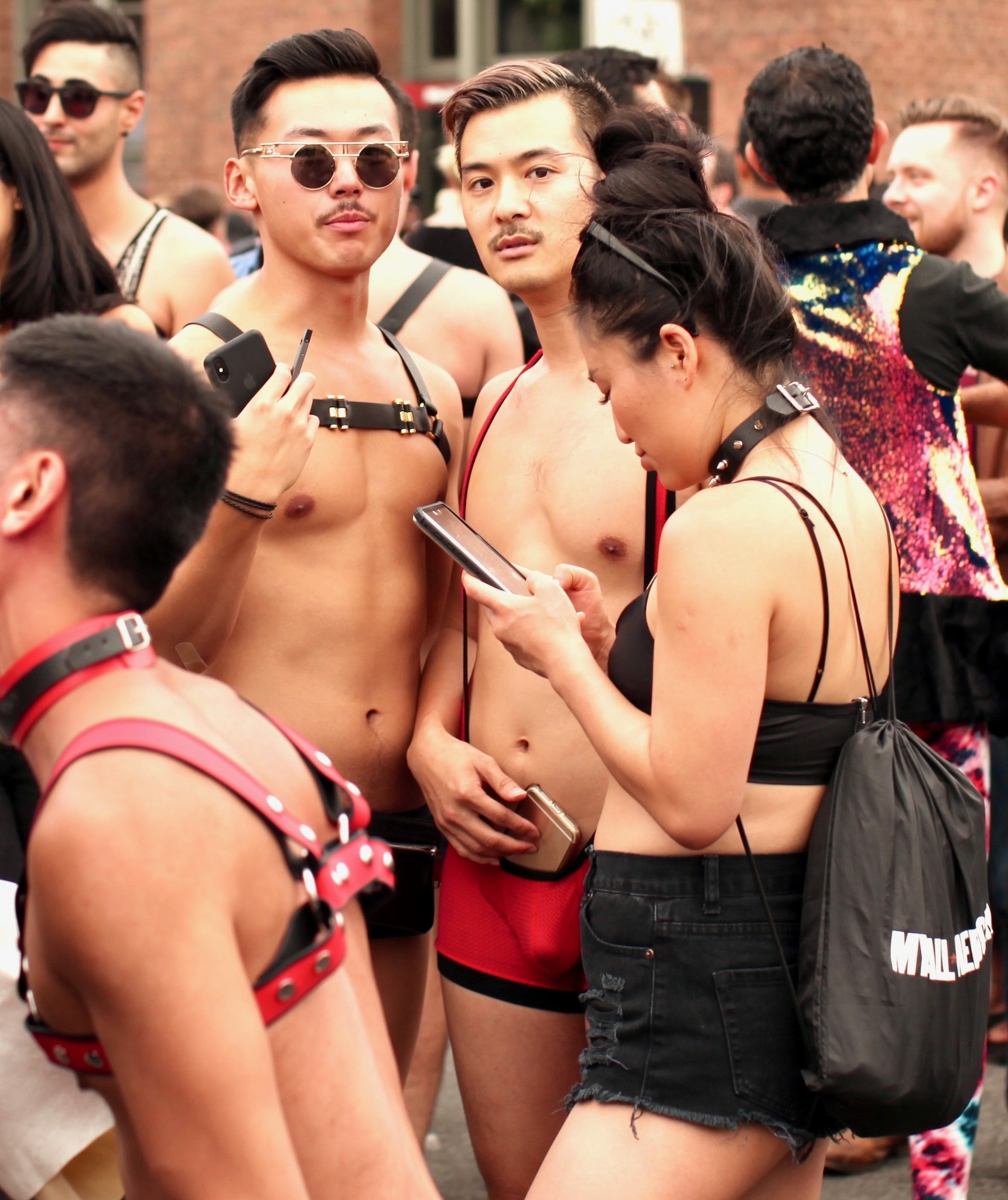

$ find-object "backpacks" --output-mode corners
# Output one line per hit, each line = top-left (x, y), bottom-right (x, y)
(734, 476), (990, 1137)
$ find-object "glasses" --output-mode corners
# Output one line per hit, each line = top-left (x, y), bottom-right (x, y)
(16, 80), (127, 118)
(240, 141), (409, 190)
(571, 220), (699, 336)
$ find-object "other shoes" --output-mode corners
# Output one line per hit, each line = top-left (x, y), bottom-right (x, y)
(988, 1022), (1008, 1043)
(825, 1128), (909, 1173)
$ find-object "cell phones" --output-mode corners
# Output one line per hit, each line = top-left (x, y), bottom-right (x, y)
(412, 501), (534, 597)
(202, 330), (276, 416)
(500, 784), (582, 871)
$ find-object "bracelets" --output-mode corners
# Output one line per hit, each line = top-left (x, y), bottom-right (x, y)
(217, 493), (273, 519)
(220, 490), (275, 511)
(222, 487), (278, 508)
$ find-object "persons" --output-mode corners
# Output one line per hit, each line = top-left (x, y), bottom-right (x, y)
(0, 0), (1007, 1200)
